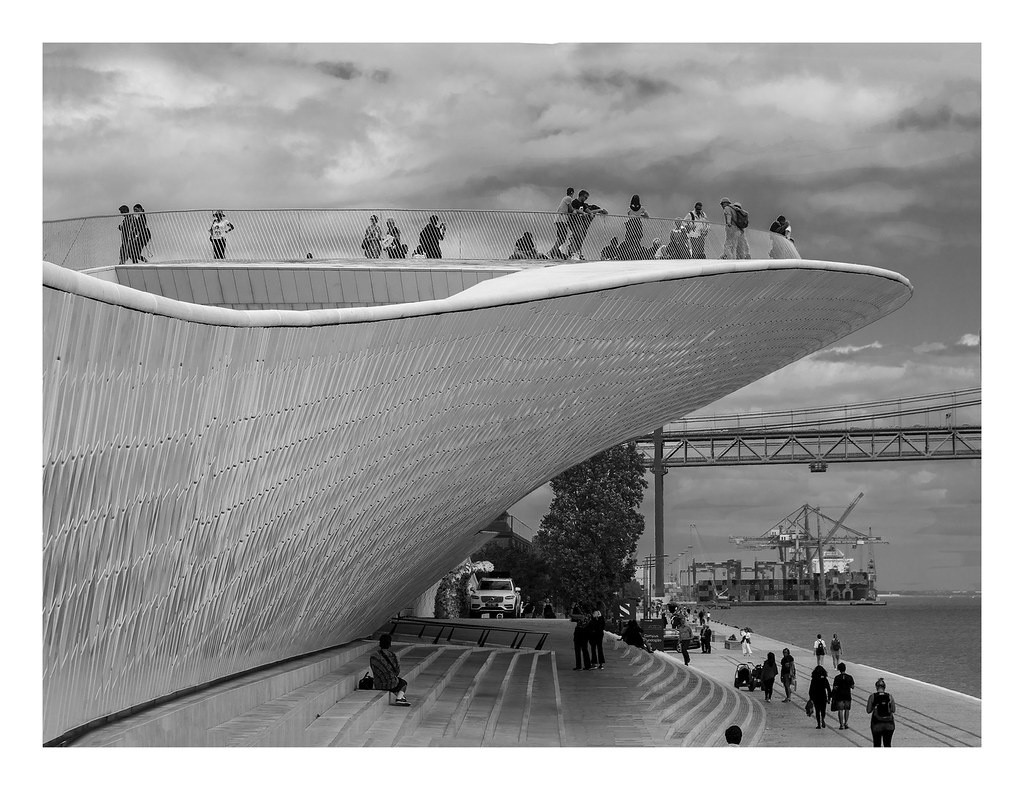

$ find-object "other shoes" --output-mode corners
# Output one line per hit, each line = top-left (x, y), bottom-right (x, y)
(816, 724), (825, 729)
(839, 726), (850, 729)
(396, 696), (411, 705)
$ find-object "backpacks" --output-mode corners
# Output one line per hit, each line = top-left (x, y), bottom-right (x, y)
(730, 206), (748, 228)
(832, 639), (840, 651)
(818, 640), (824, 655)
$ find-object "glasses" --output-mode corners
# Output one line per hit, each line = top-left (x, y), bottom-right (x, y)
(696, 209), (702, 210)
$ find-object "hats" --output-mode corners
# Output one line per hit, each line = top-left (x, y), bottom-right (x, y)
(720, 198), (731, 204)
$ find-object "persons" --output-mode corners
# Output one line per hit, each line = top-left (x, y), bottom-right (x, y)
(813, 633), (827, 666)
(867, 677), (895, 747)
(546, 187), (794, 260)
(542, 599), (556, 618)
(419, 214), (444, 259)
(724, 725), (743, 747)
(831, 662), (855, 730)
(523, 596), (535, 617)
(369, 633), (410, 705)
(648, 599), (711, 626)
(618, 618), (654, 654)
(740, 627), (752, 657)
(131, 203), (152, 264)
(699, 624), (712, 654)
(208, 209), (235, 260)
(382, 217), (401, 259)
(117, 204), (137, 264)
(809, 666), (832, 729)
(677, 619), (694, 665)
(780, 648), (797, 702)
(760, 652), (779, 703)
(831, 634), (842, 670)
(509, 232), (548, 260)
(362, 215), (382, 259)
(587, 611), (606, 669)
(573, 620), (591, 671)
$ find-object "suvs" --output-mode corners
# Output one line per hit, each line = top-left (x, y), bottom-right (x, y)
(469, 577), (523, 619)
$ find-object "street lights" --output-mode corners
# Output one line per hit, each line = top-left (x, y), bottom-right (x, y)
(640, 553), (669, 619)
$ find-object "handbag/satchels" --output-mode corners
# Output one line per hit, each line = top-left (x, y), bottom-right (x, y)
(362, 237), (372, 249)
(359, 672), (375, 690)
(805, 700), (814, 717)
(872, 710), (895, 733)
